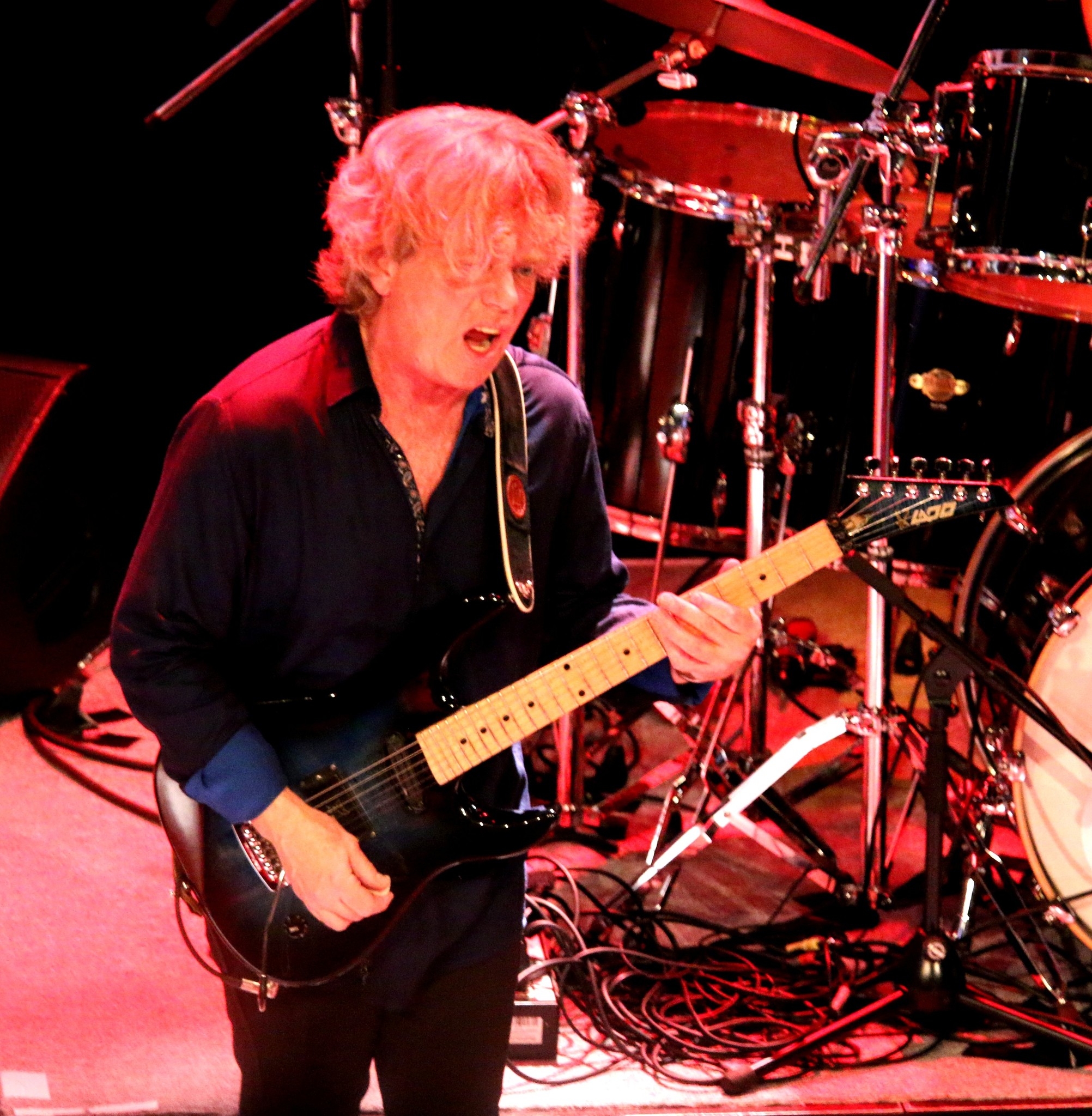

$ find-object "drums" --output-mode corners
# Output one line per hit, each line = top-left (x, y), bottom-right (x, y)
(907, 46), (1092, 325)
(516, 100), (862, 547)
(956, 425), (1092, 949)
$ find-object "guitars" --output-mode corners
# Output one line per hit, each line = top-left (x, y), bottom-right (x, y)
(154, 455), (1017, 1010)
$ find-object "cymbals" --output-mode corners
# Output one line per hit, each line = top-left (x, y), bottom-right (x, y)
(610, 0), (935, 103)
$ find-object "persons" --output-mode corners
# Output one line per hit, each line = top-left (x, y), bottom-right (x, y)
(111, 106), (759, 1115)
